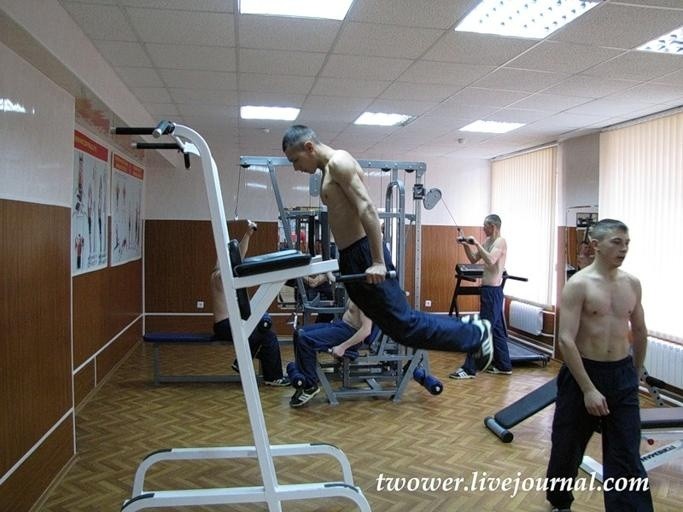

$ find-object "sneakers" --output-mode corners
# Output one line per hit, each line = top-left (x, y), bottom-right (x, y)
(231, 359), (319, 407)
(448, 314), (512, 379)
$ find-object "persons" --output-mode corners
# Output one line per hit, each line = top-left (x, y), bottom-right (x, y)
(574, 239), (597, 271)
(545, 218), (656, 512)
(209, 221), (292, 389)
(289, 295), (374, 410)
(301, 272), (336, 322)
(281, 123), (497, 375)
(448, 213), (514, 381)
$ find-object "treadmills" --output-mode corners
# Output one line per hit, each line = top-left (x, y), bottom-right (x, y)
(448, 263), (551, 366)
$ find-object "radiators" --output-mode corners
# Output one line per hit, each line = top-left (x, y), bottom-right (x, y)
(509, 300), (543, 336)
(643, 336), (683, 391)
